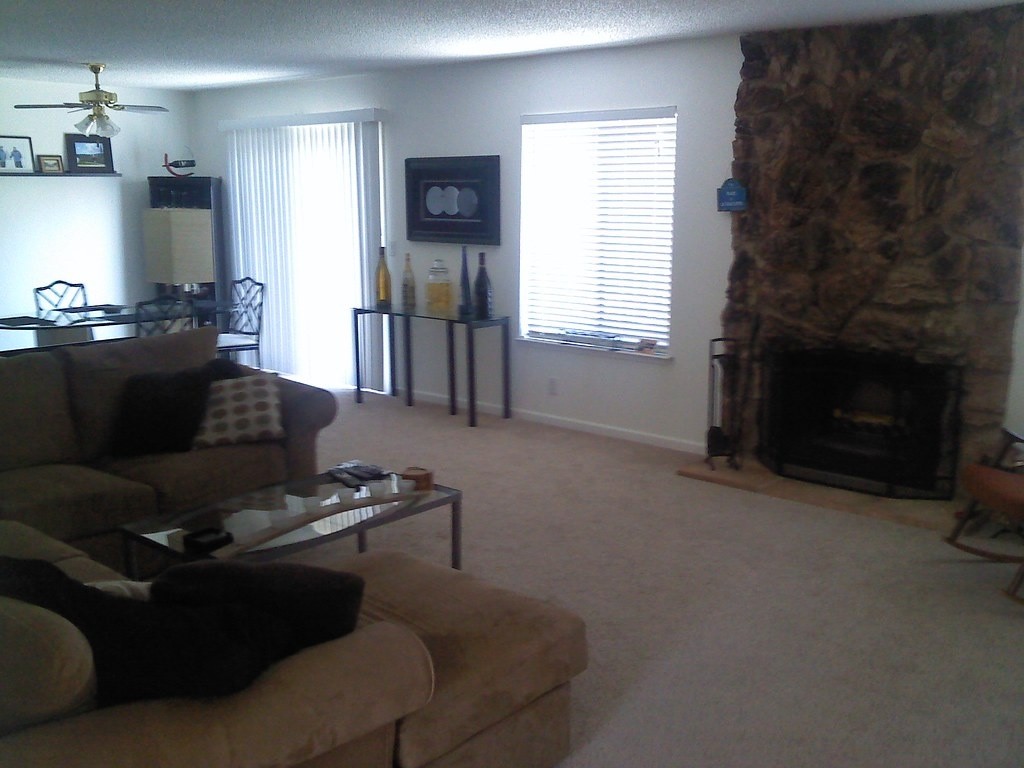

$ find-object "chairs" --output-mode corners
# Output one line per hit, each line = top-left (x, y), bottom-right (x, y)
(32, 280), (94, 347)
(944, 425), (1024, 594)
(133, 295), (195, 341)
(214, 275), (263, 369)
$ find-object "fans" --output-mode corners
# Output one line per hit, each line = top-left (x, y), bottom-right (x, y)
(14, 62), (170, 115)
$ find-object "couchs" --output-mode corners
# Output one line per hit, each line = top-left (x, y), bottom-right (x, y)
(0, 520), (589, 768)
(0, 322), (337, 573)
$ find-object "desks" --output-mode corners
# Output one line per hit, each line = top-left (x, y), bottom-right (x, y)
(0, 303), (243, 347)
(351, 299), (512, 429)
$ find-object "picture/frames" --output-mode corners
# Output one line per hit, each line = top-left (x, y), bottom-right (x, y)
(403, 154), (502, 246)
(0, 135), (36, 174)
(37, 154), (64, 175)
(64, 134), (114, 175)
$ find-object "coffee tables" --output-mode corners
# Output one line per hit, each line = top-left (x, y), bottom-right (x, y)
(119, 459), (464, 581)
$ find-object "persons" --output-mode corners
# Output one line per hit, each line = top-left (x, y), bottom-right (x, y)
(10, 147), (23, 168)
(0, 146), (7, 168)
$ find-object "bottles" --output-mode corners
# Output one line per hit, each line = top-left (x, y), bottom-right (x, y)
(475, 252), (493, 320)
(426, 260), (451, 315)
(156, 189), (203, 209)
(162, 160), (196, 168)
(400, 253), (416, 312)
(376, 246), (392, 311)
(159, 283), (212, 301)
(456, 245), (475, 321)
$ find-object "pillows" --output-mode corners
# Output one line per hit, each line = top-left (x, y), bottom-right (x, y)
(0, 552), (367, 710)
(192, 370), (287, 449)
(105, 364), (213, 459)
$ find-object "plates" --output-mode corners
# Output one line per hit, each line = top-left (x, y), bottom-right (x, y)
(457, 188), (478, 217)
(441, 185), (460, 215)
(425, 186), (444, 215)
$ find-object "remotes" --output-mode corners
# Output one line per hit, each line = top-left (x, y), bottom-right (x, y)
(329, 459), (387, 488)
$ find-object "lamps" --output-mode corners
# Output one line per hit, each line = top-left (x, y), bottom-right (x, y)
(72, 101), (122, 139)
